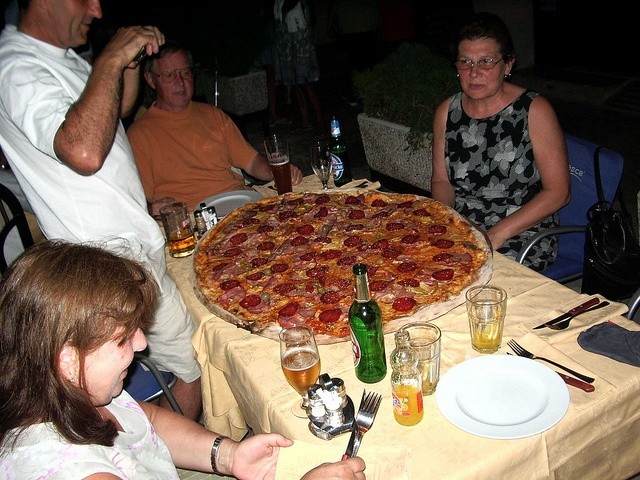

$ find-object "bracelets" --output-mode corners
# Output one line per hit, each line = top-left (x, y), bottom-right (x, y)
(210, 434), (229, 474)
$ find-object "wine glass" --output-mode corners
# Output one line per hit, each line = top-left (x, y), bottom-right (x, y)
(278, 325), (322, 418)
(309, 142), (334, 190)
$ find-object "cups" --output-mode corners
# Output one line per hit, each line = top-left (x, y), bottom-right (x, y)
(464, 285), (508, 354)
(397, 323), (441, 397)
(159, 202), (198, 258)
(263, 134), (292, 196)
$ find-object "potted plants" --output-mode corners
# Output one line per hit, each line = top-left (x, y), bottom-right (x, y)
(199, 36), (270, 116)
(355, 42), (459, 195)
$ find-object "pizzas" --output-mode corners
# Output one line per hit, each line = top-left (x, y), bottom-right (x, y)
(193, 188), (487, 336)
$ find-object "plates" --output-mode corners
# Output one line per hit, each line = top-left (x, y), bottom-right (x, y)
(434, 354), (570, 439)
(196, 190), (263, 219)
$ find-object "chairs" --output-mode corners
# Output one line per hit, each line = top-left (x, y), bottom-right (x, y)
(134, 93), (273, 217)
(0, 178), (184, 417)
(516, 132), (626, 283)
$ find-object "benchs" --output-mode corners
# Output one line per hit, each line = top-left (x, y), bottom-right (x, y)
(510, 65), (640, 121)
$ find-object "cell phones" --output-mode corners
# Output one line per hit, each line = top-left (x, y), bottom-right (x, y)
(133, 48), (147, 62)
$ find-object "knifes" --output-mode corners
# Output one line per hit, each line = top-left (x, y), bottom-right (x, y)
(532, 297), (600, 330)
(341, 389), (366, 462)
(507, 352), (594, 392)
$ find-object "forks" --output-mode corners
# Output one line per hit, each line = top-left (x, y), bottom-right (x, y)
(350, 390), (383, 459)
(507, 339), (595, 383)
(544, 301), (610, 330)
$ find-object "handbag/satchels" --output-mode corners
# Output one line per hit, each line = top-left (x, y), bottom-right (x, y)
(581, 146), (639, 300)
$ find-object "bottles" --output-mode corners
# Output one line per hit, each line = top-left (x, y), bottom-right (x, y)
(346, 263), (389, 382)
(330, 111), (352, 187)
(390, 331), (425, 428)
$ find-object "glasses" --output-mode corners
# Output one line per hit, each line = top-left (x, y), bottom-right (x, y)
(455, 55), (504, 70)
(150, 68), (191, 83)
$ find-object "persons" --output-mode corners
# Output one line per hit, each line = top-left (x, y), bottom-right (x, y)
(127, 40), (303, 225)
(428, 28), (573, 269)
(0, 0), (205, 424)
(0, 236), (367, 480)
